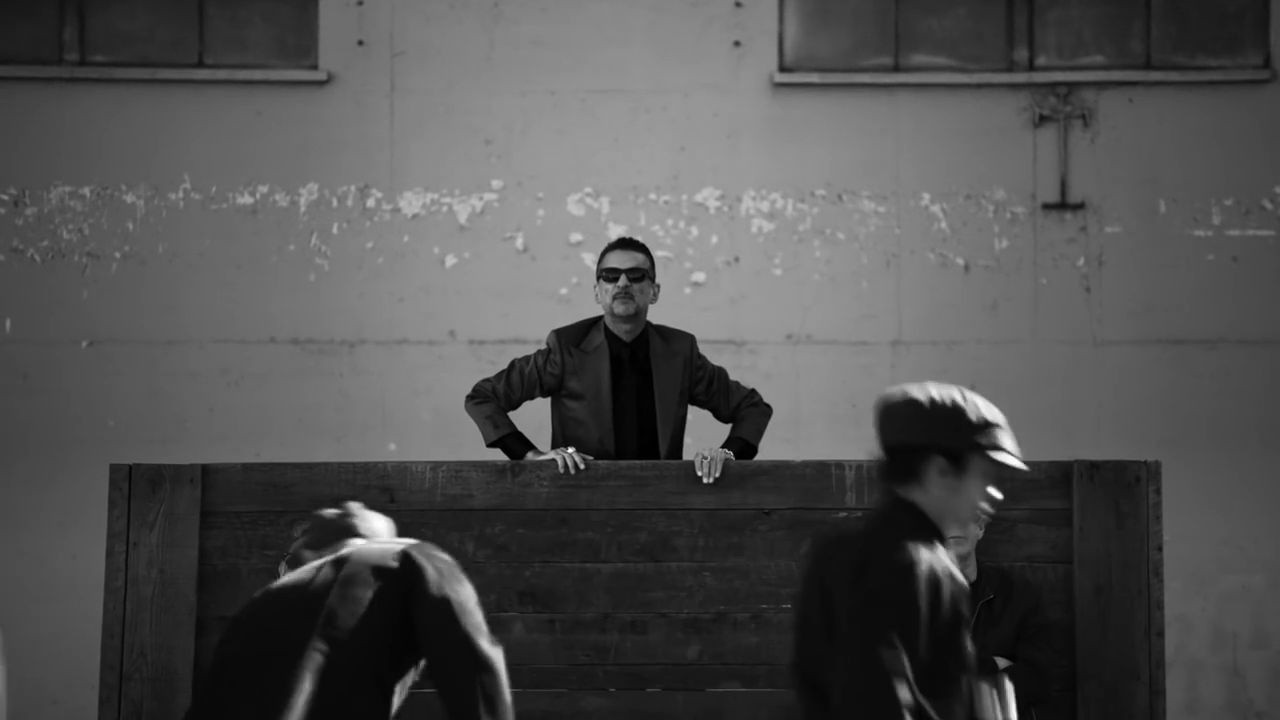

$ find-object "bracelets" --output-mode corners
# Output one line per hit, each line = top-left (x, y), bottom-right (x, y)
(719, 449), (734, 460)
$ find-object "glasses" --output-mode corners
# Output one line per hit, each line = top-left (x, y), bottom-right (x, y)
(597, 267), (655, 283)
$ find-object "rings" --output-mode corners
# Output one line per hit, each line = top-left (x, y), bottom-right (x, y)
(566, 446), (576, 453)
(701, 455), (710, 463)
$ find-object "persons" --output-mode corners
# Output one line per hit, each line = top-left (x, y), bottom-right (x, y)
(792, 382), (1033, 720)
(464, 237), (773, 484)
(185, 497), (516, 720)
(946, 517), (1053, 720)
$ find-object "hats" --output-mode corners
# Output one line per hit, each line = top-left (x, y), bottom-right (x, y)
(877, 382), (1030, 471)
(221, 499), (435, 676)
